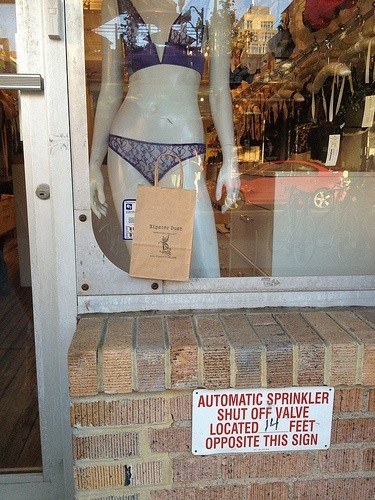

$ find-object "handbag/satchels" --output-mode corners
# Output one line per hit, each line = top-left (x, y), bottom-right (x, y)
(127, 151), (197, 283)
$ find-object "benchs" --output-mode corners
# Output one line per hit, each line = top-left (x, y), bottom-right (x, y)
(67, 308), (374, 500)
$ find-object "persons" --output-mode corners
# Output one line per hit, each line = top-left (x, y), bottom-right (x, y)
(88, 0), (241, 278)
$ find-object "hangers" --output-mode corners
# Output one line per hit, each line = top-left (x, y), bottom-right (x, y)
(219, 7), (375, 126)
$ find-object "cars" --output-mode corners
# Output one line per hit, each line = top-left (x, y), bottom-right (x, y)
(213, 159), (349, 209)
